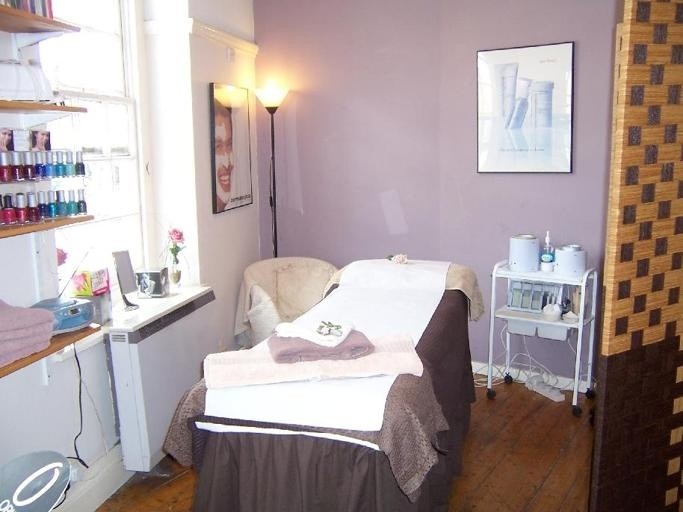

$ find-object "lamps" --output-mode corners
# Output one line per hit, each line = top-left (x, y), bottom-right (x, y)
(255, 82), (292, 256)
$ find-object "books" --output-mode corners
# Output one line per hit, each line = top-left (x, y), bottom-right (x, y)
(0, 0), (53, 19)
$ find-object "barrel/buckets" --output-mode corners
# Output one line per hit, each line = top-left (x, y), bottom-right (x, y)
(508, 234), (540, 273)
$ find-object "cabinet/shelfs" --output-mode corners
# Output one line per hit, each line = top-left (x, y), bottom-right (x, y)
(0, 3), (104, 380)
(486, 259), (599, 417)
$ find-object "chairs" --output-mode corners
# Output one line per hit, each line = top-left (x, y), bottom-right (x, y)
(241, 256), (337, 347)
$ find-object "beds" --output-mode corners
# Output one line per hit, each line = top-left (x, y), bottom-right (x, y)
(193, 258), (473, 511)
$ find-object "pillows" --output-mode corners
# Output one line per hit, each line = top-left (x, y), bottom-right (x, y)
(320, 259), (484, 321)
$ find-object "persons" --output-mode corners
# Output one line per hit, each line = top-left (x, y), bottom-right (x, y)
(215, 98), (234, 213)
(0, 128), (12, 152)
(32, 131), (50, 151)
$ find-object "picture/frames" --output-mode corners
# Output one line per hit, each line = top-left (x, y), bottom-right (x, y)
(208, 79), (253, 215)
(475, 40), (575, 176)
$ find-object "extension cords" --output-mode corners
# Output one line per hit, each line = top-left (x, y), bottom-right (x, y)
(524, 375), (567, 404)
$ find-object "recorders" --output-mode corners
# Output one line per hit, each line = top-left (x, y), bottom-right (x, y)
(28, 295), (97, 337)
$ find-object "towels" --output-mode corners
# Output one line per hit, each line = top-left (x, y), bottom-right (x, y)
(267, 330), (374, 363)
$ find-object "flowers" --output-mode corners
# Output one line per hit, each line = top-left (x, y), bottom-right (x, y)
(315, 320), (342, 337)
(384, 254), (407, 265)
(164, 225), (185, 285)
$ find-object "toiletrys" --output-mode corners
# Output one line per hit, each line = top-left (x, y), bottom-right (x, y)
(540, 230), (554, 260)
(0, 152), (87, 225)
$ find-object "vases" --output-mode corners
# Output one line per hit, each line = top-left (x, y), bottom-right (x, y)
(168, 253), (182, 284)
